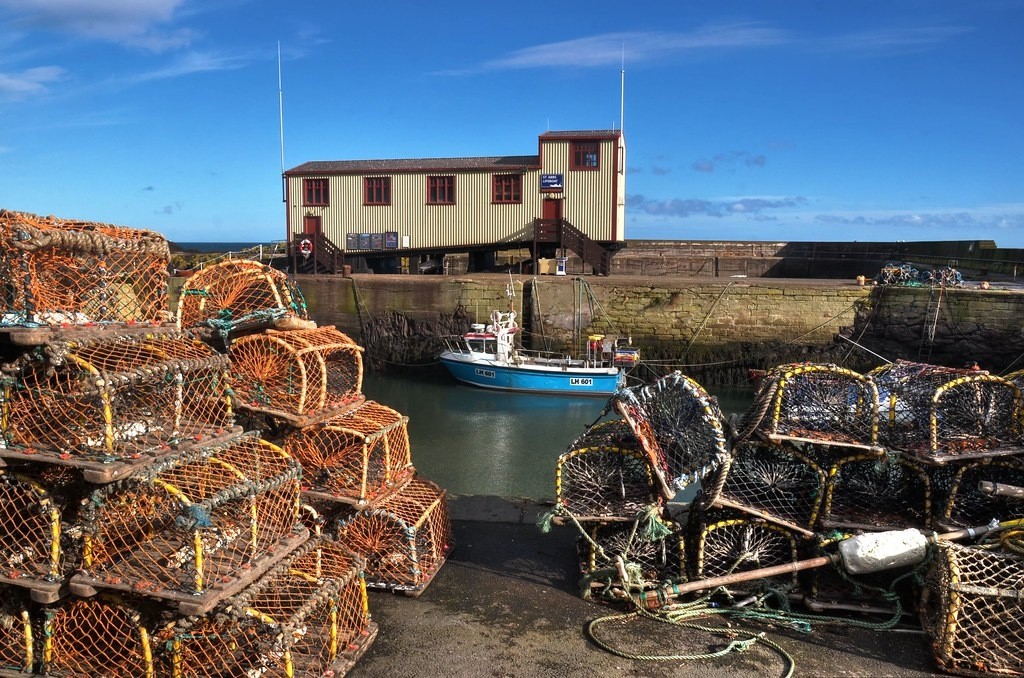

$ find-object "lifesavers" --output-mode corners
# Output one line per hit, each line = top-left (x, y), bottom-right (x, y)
(593, 334), (605, 338)
(588, 336), (601, 341)
(505, 321), (518, 334)
(492, 324), (501, 335)
(490, 312), (502, 321)
(299, 239), (312, 254)
(509, 310), (516, 318)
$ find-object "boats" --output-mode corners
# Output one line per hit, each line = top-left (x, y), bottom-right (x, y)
(438, 277), (639, 396)
